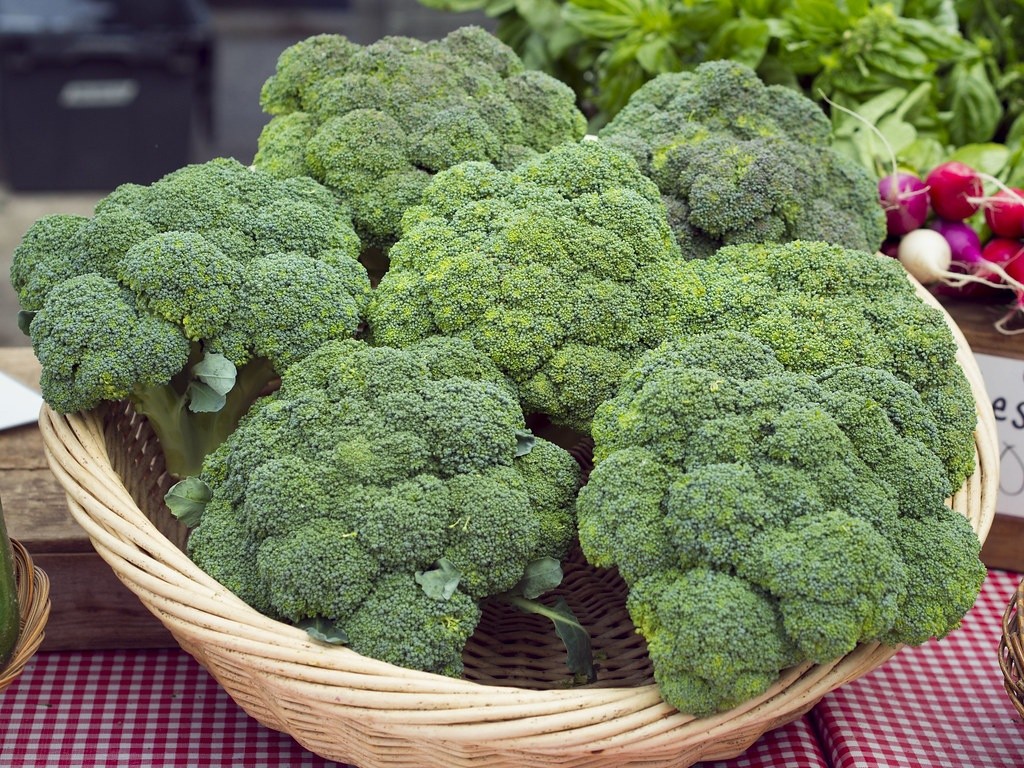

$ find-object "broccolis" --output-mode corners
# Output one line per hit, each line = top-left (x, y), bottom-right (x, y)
(12, 24), (984, 715)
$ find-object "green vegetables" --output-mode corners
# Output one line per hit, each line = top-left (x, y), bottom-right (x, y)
(419, 0), (1024, 240)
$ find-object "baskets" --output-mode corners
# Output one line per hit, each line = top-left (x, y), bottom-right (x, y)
(1000, 580), (1024, 719)
(0, 535), (52, 695)
(38, 250), (999, 768)
